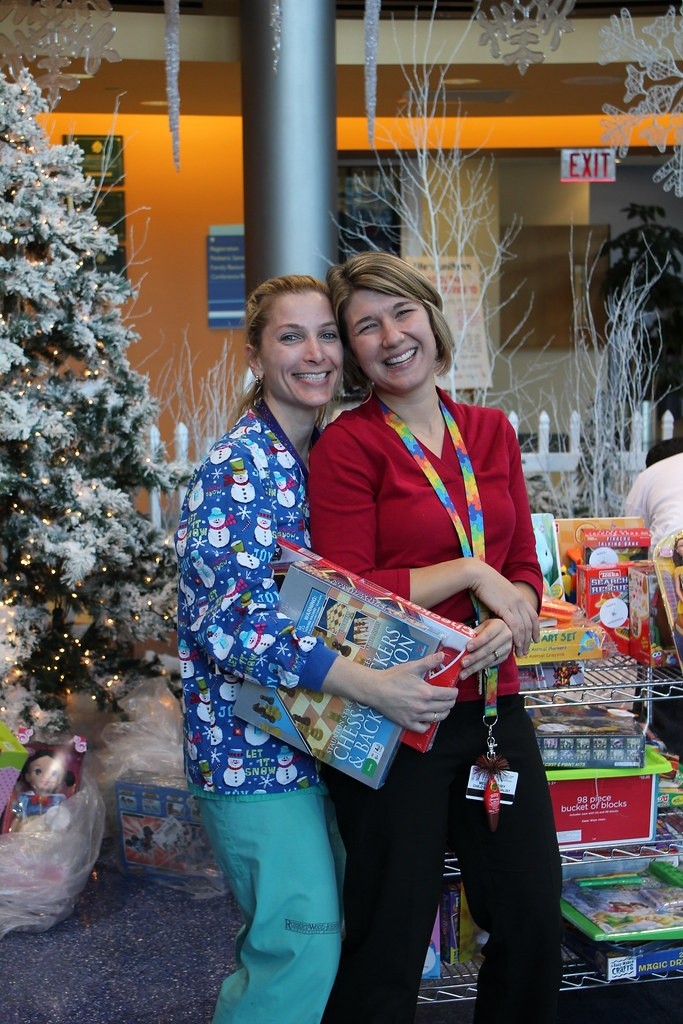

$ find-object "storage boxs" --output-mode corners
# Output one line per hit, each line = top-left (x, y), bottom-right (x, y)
(116, 770), (223, 883)
(270, 537), (478, 753)
(232, 561), (441, 789)
(515, 513), (683, 673)
(530, 707), (683, 981)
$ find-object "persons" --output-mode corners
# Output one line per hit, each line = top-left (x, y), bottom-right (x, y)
(622, 437), (683, 561)
(174, 275), (455, 1024)
(303, 251), (561, 1023)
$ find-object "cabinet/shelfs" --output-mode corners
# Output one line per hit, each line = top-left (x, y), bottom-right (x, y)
(416, 658), (683, 1004)
